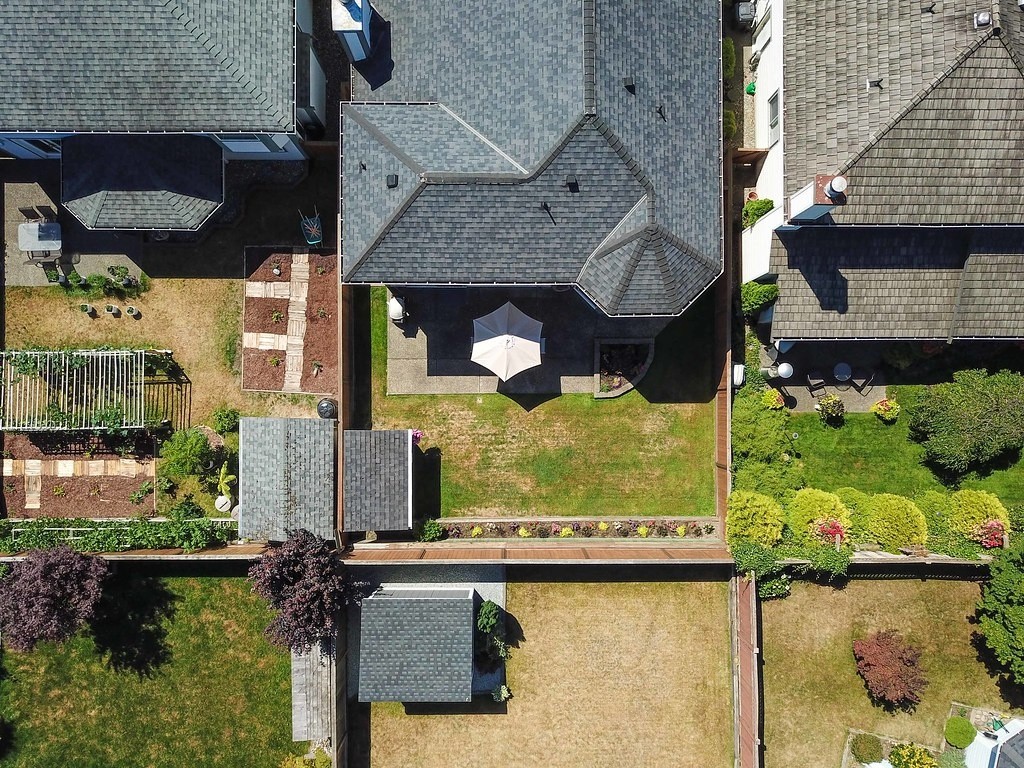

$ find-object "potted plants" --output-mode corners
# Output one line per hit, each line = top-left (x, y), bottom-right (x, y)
(115, 275), (130, 285)
(125, 306), (138, 316)
(67, 270), (85, 288)
(46, 270), (65, 283)
(201, 459), (214, 469)
(81, 303), (92, 313)
(105, 304), (118, 314)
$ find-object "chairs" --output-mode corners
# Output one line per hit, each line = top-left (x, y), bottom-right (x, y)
(851, 369), (875, 396)
(470, 337), (474, 353)
(540, 338), (546, 354)
(26, 251), (62, 268)
(806, 374), (827, 397)
(36, 205), (57, 223)
(17, 206), (43, 223)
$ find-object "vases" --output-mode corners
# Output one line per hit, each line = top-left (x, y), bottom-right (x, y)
(746, 191), (759, 202)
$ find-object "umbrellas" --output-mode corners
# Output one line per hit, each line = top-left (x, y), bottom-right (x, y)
(470, 301), (543, 382)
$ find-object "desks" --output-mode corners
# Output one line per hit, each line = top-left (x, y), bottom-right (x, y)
(834, 362), (851, 391)
(17, 223), (62, 250)
